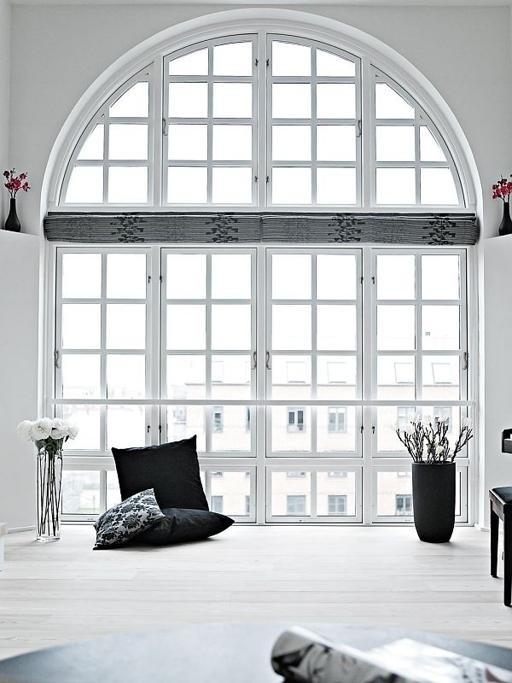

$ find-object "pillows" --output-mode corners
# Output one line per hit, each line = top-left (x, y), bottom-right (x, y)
(134, 508), (235, 547)
(92, 488), (165, 550)
(111, 434), (209, 510)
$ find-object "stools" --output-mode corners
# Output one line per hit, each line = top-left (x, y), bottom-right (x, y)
(489, 486), (512, 606)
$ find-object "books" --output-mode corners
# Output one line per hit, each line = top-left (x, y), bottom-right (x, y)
(269, 622), (511, 682)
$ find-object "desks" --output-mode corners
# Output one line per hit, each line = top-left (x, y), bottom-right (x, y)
(0, 625), (512, 683)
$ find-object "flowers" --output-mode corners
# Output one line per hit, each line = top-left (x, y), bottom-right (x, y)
(492, 174), (512, 202)
(3, 168), (30, 198)
(391, 414), (474, 464)
(15, 417), (79, 537)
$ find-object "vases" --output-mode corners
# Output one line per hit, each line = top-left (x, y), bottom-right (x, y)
(5, 199), (21, 232)
(36, 452), (63, 543)
(412, 461), (456, 543)
(499, 202), (512, 236)
